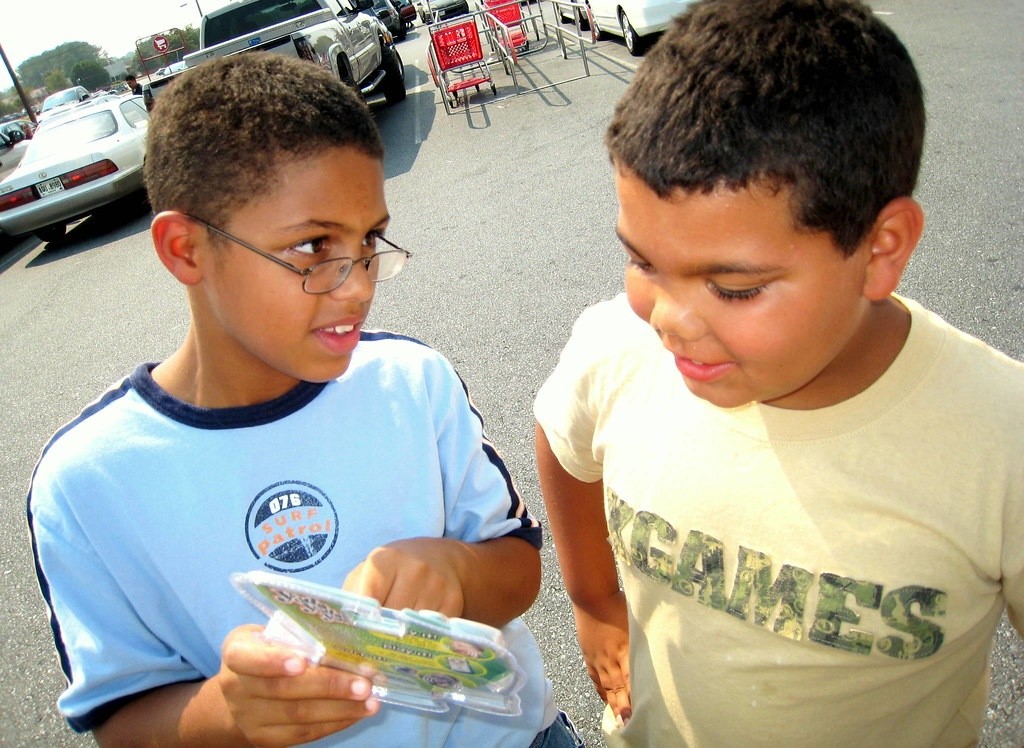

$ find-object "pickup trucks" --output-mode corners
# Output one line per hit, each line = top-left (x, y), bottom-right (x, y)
(144, 0), (406, 120)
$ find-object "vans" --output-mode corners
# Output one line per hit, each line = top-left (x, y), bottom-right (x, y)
(554, 0), (695, 57)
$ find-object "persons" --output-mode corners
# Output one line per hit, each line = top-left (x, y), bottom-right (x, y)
(533, 0), (1024, 748)
(21, 122), (34, 140)
(25, 50), (586, 748)
(125, 75), (143, 95)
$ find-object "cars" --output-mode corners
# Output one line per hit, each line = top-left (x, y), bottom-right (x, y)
(0, 94), (165, 242)
(0, 120), (38, 173)
(391, 0), (418, 26)
(416, 0), (469, 25)
(39, 85), (92, 120)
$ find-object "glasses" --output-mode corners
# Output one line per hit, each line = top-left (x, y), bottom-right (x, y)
(182, 213), (413, 295)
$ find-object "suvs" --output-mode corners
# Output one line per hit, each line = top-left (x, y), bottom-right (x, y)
(372, 0), (406, 40)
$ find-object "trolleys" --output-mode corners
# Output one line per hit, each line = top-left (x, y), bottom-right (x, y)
(427, 13), (496, 107)
(480, 0), (529, 53)
